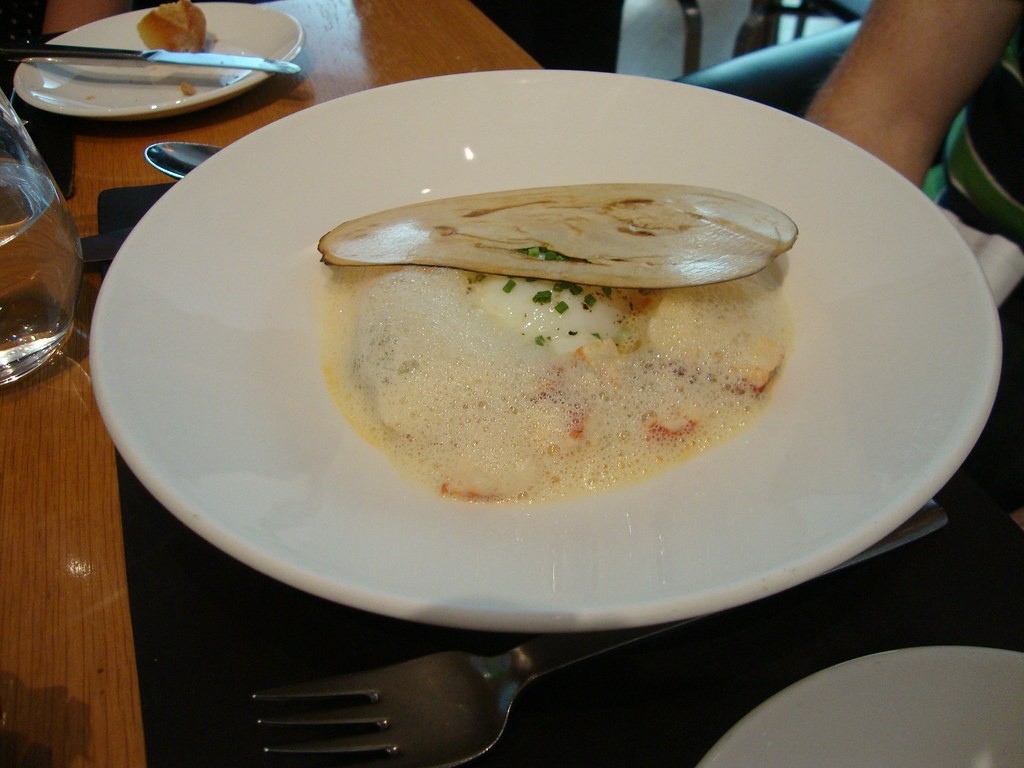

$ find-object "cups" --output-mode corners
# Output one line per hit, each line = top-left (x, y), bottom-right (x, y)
(1, 84), (83, 387)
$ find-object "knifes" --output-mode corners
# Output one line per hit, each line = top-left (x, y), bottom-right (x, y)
(1, 39), (302, 75)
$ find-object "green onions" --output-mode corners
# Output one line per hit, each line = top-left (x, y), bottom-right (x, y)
(503, 248), (617, 345)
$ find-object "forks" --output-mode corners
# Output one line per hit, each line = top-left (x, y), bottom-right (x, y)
(248, 495), (949, 767)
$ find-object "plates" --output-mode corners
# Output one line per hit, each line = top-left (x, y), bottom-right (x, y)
(13, 1), (306, 121)
(684, 646), (1024, 768)
(84, 67), (1003, 636)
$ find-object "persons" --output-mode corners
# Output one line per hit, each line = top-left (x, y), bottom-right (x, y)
(808, 0), (1024, 310)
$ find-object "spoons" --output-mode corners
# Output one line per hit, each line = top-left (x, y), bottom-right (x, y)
(143, 138), (226, 181)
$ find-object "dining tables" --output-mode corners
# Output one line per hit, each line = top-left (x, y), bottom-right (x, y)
(2, 0), (1024, 767)
